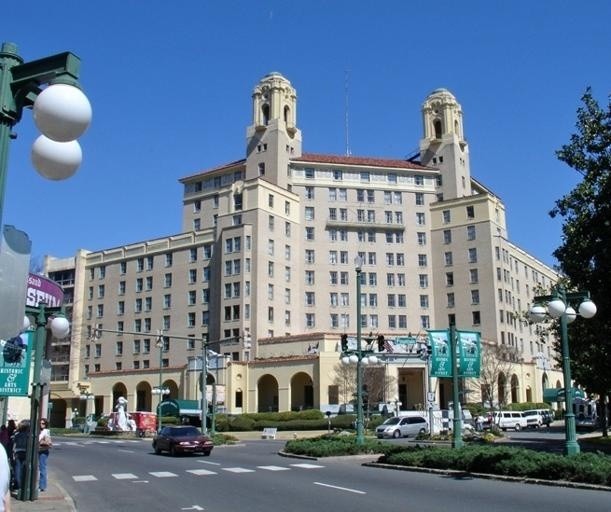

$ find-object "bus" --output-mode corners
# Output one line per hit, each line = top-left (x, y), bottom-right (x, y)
(572, 397), (598, 431)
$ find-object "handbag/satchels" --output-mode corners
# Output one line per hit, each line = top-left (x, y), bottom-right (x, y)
(37, 443), (49, 455)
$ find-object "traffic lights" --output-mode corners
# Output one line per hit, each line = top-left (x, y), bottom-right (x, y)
(378, 335), (385, 352)
(241, 330), (252, 351)
(341, 334), (348, 351)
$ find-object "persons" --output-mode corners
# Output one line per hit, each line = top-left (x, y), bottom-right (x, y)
(0, 418), (52, 512)
(475, 409), (493, 431)
(84, 416), (137, 433)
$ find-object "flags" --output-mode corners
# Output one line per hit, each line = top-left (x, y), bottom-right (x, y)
(383, 341), (394, 353)
(416, 330), (428, 343)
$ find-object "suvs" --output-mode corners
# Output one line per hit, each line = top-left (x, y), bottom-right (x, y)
(524, 410), (543, 428)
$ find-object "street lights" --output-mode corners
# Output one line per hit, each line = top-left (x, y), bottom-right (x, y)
(19, 300), (72, 503)
(526, 277), (598, 458)
(72, 407), (79, 428)
(152, 384), (172, 432)
(153, 329), (166, 403)
(0, 37), (93, 247)
(351, 254), (366, 445)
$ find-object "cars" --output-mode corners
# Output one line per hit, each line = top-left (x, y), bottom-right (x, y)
(375, 416), (429, 439)
(152, 425), (214, 457)
(80, 410), (156, 435)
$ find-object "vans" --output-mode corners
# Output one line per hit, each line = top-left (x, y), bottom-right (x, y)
(483, 411), (527, 432)
(537, 408), (554, 427)
